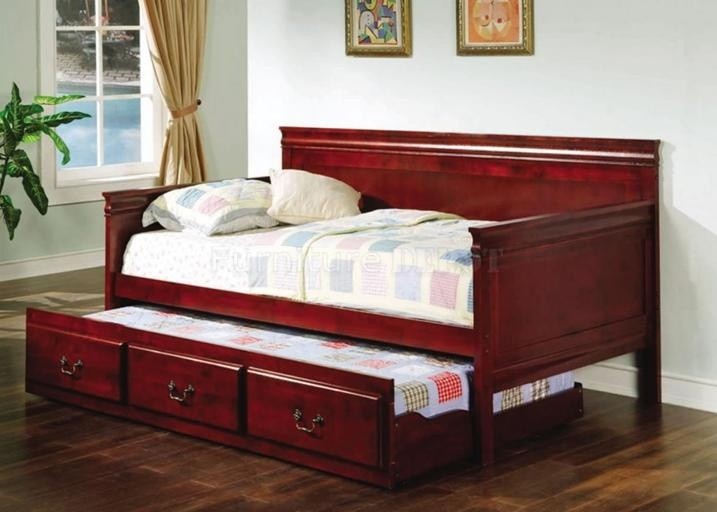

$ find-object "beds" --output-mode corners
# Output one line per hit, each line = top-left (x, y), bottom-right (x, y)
(24, 126), (661, 488)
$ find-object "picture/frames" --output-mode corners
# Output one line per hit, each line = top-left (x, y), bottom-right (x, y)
(456, 0), (536, 56)
(345, 0), (412, 56)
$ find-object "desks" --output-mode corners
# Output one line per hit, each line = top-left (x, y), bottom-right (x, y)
(80, 23), (133, 69)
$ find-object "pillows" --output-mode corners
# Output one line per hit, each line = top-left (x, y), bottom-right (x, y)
(269, 166), (366, 226)
(138, 179), (278, 236)
